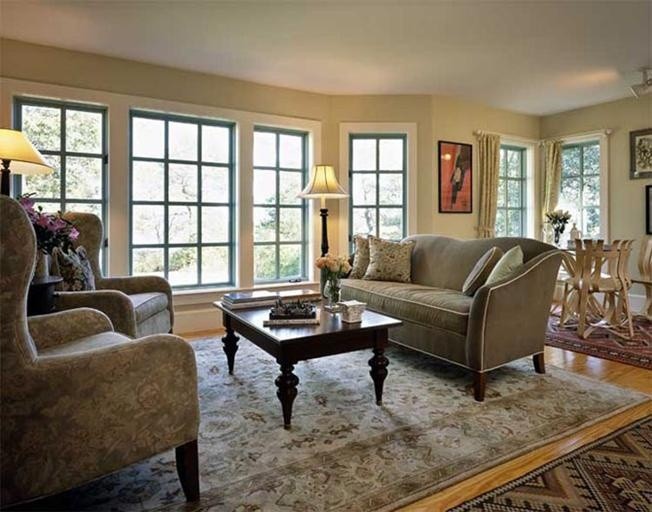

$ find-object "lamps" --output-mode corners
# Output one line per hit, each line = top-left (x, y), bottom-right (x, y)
(629, 65), (651, 99)
(296, 165), (350, 299)
(0, 129), (55, 197)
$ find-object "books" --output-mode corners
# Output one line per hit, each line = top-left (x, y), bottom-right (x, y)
(220, 288), (324, 325)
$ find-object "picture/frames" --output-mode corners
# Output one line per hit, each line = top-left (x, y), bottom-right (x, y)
(629, 128), (652, 179)
(438, 139), (472, 213)
(645, 185), (651, 235)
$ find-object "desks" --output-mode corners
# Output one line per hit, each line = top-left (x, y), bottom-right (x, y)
(27, 275), (63, 316)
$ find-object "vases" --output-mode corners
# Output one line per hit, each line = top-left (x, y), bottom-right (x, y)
(553, 229), (561, 247)
(34, 255), (49, 281)
(322, 279), (343, 311)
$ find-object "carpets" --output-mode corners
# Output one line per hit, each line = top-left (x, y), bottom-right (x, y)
(544, 299), (652, 371)
(449, 413), (652, 512)
(2, 325), (651, 512)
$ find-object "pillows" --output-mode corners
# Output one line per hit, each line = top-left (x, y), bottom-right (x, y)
(352, 234), (370, 279)
(462, 246), (504, 296)
(362, 234), (416, 283)
(54, 243), (95, 292)
(486, 244), (524, 287)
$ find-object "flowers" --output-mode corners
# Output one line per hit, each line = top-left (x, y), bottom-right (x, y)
(543, 208), (571, 235)
(15, 193), (79, 255)
(314, 252), (351, 303)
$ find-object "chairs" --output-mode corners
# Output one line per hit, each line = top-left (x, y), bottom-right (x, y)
(548, 235), (652, 339)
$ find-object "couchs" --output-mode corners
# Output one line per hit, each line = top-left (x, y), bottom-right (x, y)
(0, 195), (198, 504)
(38, 211), (174, 337)
(343, 235), (564, 401)
